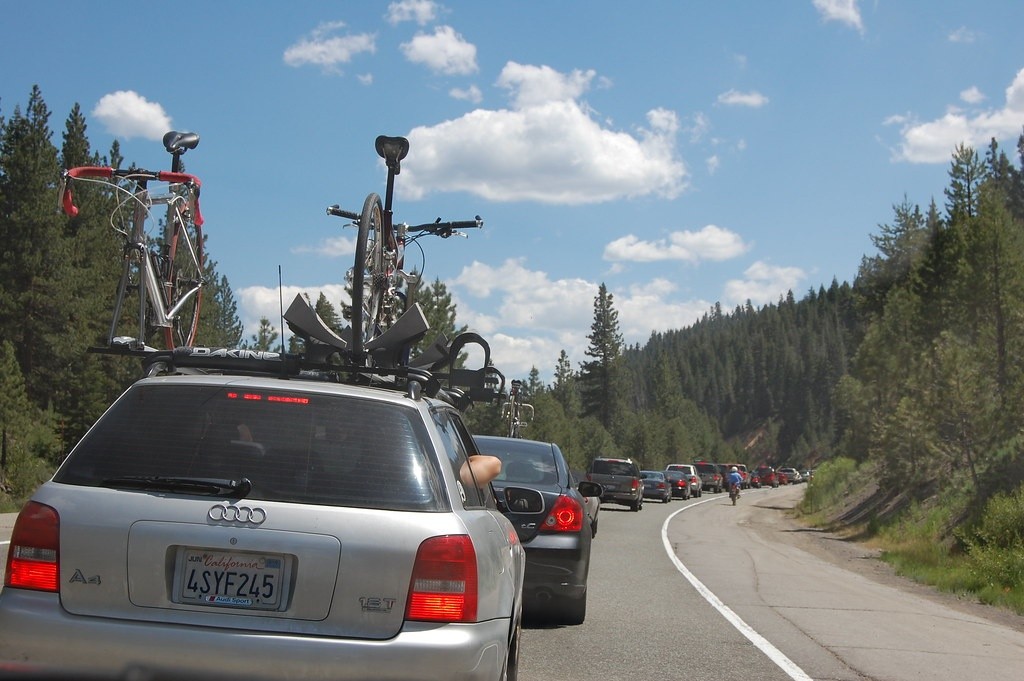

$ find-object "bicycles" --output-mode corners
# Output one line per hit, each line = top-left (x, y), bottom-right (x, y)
(324, 134), (485, 374)
(500, 380), (536, 436)
(56, 128), (214, 354)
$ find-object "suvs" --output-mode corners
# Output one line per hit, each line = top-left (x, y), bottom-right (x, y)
(586, 456), (648, 511)
(666, 464), (703, 498)
(693, 461), (723, 493)
(0, 342), (547, 680)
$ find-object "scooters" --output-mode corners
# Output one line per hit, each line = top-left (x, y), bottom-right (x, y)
(728, 482), (741, 506)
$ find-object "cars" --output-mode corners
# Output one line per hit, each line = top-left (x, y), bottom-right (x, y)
(663, 471), (692, 499)
(717, 462), (813, 490)
(469, 434), (603, 625)
(570, 469), (601, 538)
(637, 471), (673, 504)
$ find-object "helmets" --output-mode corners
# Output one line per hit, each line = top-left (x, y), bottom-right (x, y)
(731, 467), (738, 472)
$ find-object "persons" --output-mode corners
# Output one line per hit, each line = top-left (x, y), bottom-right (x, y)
(470, 451), (502, 489)
(727, 466), (743, 497)
